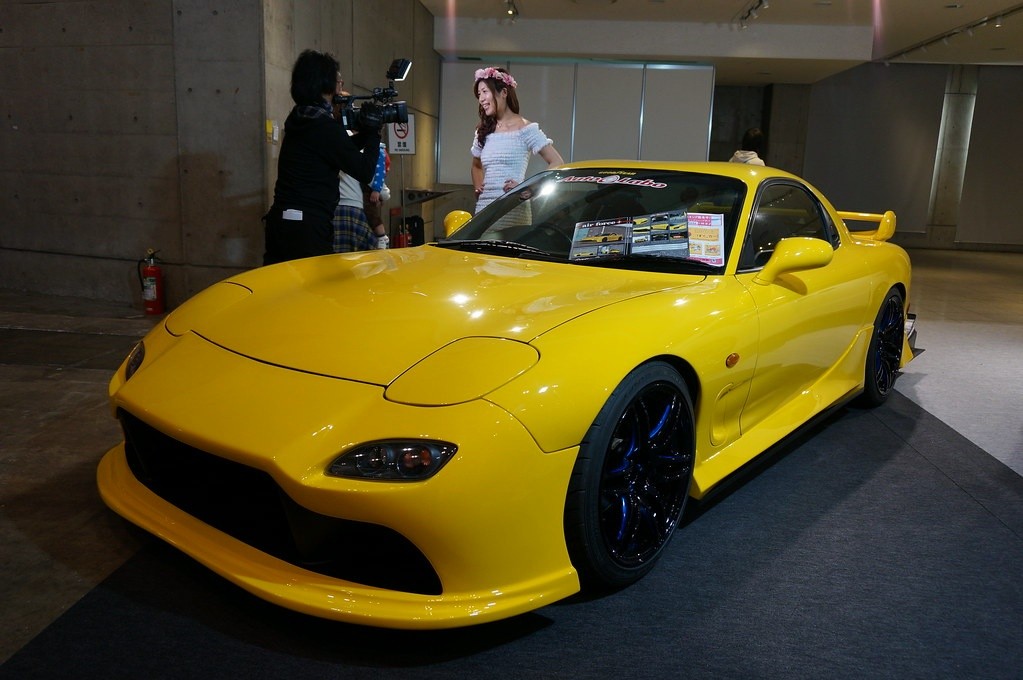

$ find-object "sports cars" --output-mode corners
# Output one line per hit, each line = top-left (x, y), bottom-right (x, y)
(95, 159), (928, 632)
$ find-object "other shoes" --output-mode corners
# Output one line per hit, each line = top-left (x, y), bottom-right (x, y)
(377, 235), (389, 250)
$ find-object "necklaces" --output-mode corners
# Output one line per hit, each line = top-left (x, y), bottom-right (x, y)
(496, 120), (505, 128)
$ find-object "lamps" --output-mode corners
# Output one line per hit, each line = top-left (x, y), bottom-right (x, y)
(507, 2), (515, 15)
(386, 58), (412, 89)
(995, 14), (1003, 28)
(740, 0), (770, 29)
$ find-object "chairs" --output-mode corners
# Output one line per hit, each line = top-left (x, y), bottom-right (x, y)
(578, 192), (647, 226)
(690, 183), (755, 272)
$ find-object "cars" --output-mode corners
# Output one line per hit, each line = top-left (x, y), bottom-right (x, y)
(573, 250), (594, 258)
(580, 231), (624, 243)
(600, 248), (620, 255)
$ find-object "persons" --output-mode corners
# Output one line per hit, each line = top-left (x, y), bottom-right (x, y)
(729, 125), (767, 167)
(261, 47), (392, 269)
(470, 66), (563, 239)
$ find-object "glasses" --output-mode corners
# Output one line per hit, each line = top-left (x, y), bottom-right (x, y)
(337, 81), (344, 87)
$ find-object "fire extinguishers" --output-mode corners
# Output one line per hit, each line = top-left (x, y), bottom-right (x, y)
(136, 248), (166, 319)
(397, 225), (411, 248)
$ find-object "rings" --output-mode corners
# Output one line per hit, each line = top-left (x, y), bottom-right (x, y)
(474, 189), (478, 192)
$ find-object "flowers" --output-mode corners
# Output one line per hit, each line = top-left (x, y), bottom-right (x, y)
(473, 68), (518, 87)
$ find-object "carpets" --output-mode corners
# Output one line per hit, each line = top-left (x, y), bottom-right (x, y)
(0, 384), (1023, 680)
